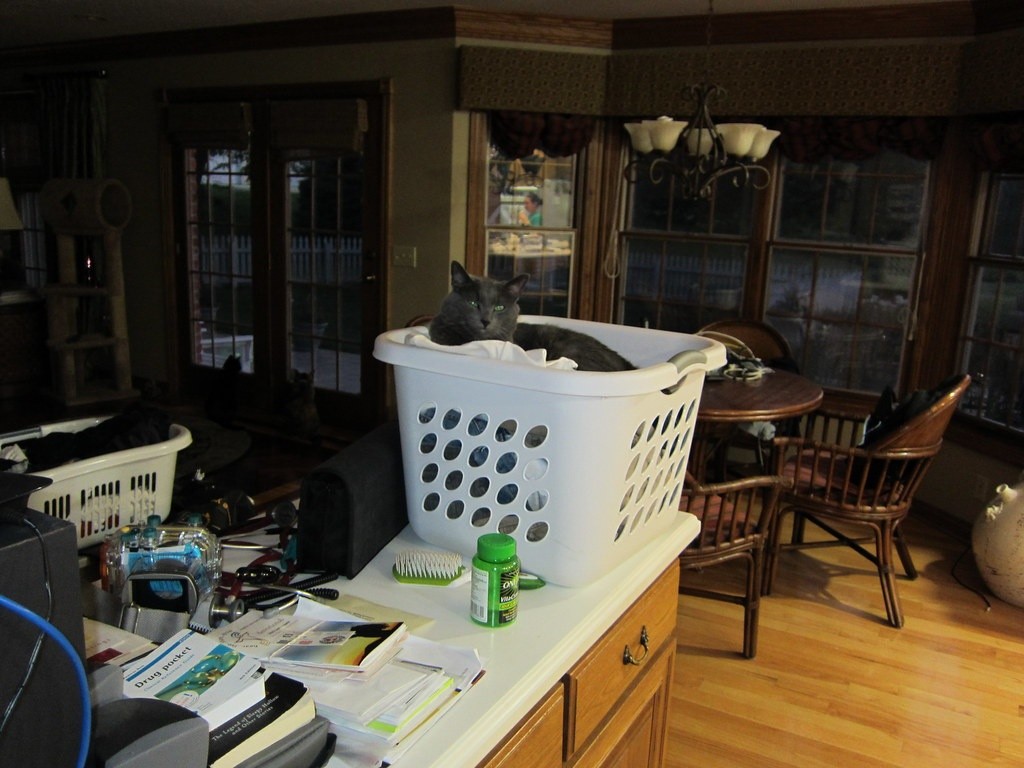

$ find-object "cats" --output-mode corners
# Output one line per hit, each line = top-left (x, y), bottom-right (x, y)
(428, 260), (682, 449)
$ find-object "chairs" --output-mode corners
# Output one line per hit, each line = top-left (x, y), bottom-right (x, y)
(678, 316), (973, 660)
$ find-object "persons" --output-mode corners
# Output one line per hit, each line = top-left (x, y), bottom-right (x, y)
(518, 190), (544, 228)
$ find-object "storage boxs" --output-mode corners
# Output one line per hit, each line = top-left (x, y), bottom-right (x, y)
(370, 315), (728, 592)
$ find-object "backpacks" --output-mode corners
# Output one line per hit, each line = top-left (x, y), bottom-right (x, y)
(840, 385), (942, 490)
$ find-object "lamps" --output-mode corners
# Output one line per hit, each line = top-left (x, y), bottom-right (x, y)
(0, 177), (27, 292)
(490, 148), (546, 196)
(622, 0), (781, 208)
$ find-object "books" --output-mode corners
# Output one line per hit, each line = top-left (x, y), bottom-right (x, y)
(123, 609), (486, 768)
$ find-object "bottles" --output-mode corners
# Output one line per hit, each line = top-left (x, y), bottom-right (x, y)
(100, 513), (212, 590)
(471, 533), (520, 627)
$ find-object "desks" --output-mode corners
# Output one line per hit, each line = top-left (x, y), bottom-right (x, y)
(688, 367), (825, 507)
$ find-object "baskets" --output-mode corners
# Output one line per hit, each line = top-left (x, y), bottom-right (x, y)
(0, 415), (193, 550)
(372, 314), (728, 587)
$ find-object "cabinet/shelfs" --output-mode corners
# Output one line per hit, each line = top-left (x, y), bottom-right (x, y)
(0, 290), (46, 436)
(315, 504), (702, 768)
